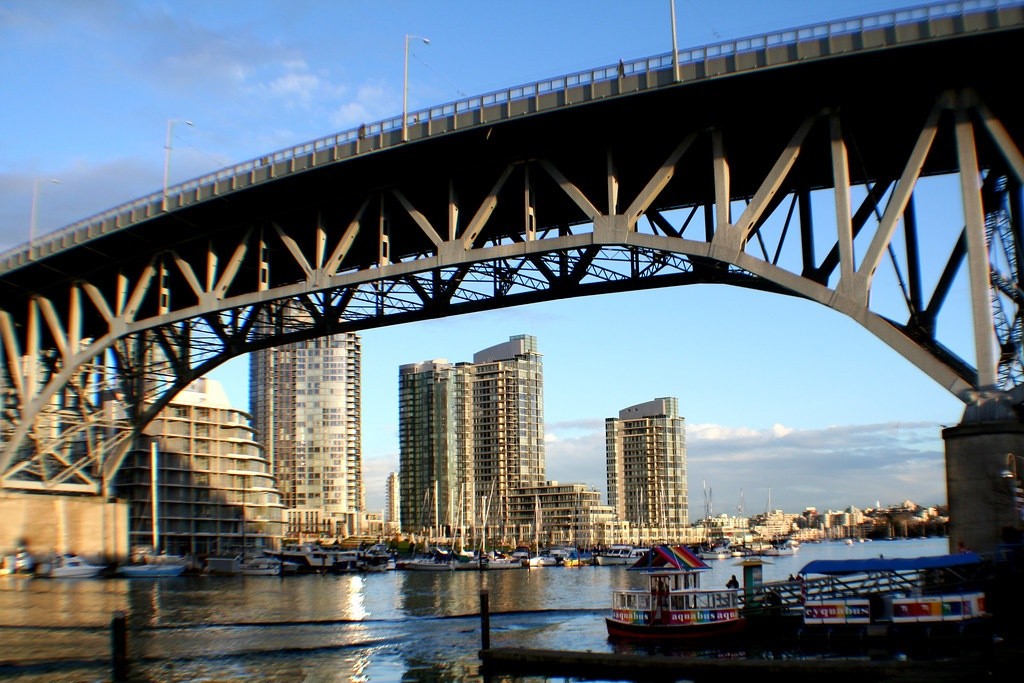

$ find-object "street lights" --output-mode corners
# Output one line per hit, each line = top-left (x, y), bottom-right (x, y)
(25, 173), (61, 261)
(399, 32), (433, 143)
(161, 113), (194, 211)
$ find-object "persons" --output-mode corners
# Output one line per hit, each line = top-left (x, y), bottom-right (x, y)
(616, 59), (626, 77)
(726, 575), (739, 589)
(796, 574), (804, 583)
(414, 114), (420, 124)
(789, 574), (795, 582)
(358, 123), (366, 139)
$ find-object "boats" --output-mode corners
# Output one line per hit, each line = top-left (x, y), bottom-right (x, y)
(34, 554), (108, 578)
(115, 551), (186, 576)
(241, 475), (593, 576)
(596, 538), (997, 663)
